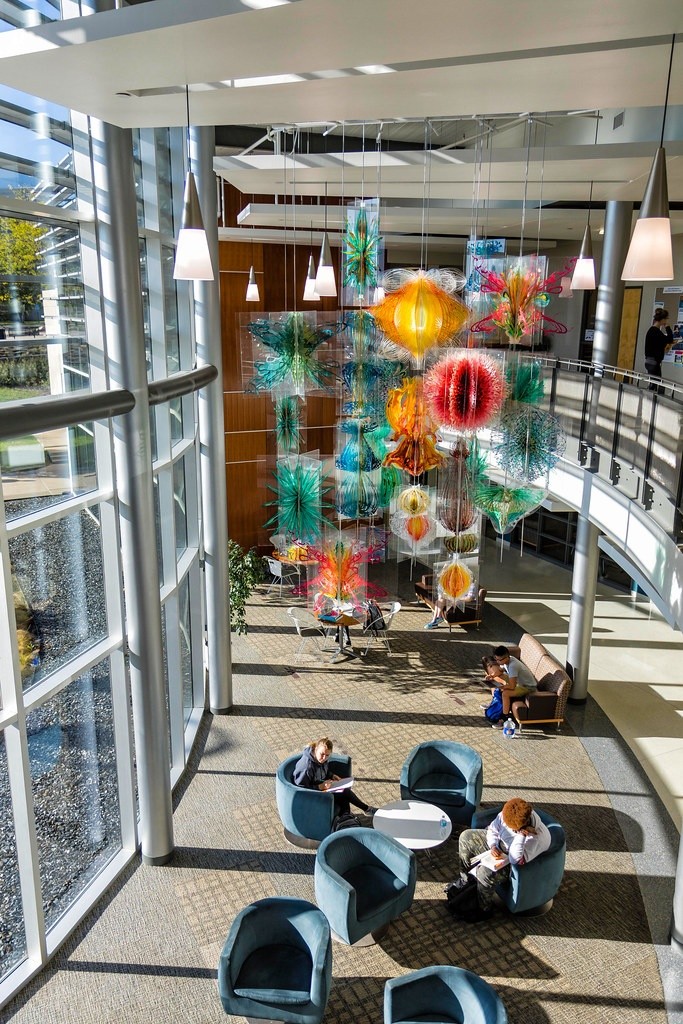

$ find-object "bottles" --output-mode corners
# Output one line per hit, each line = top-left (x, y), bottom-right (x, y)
(439, 815), (447, 840)
(503, 718), (515, 741)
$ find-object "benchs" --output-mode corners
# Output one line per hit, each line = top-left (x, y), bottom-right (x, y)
(415, 574), (487, 634)
(495, 633), (573, 733)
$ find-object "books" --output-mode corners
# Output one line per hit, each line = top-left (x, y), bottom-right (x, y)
(333, 788), (344, 793)
(470, 849), (509, 872)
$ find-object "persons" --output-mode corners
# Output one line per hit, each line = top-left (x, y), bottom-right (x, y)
(480, 646), (537, 730)
(458, 798), (551, 919)
(316, 592), (354, 651)
(425, 575), (478, 629)
(293, 736), (378, 817)
(645, 308), (674, 391)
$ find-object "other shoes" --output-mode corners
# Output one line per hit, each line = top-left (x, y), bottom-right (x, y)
(364, 806), (378, 816)
(444, 878), (467, 893)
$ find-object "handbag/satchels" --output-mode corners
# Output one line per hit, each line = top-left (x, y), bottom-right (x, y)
(363, 605), (386, 629)
(444, 880), (480, 921)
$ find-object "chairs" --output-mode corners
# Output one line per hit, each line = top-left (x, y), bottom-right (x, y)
(263, 556), (301, 598)
(361, 601), (401, 656)
(399, 741), (483, 827)
(471, 806), (566, 919)
(219, 897), (332, 1024)
(314, 827), (417, 947)
(384, 965), (508, 1024)
(287, 607), (326, 664)
(276, 753), (352, 849)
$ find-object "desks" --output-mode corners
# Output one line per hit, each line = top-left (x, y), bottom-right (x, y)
(373, 800), (452, 869)
(272, 549), (319, 597)
(314, 608), (371, 664)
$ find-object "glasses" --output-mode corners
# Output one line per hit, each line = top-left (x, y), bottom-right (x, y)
(495, 656), (508, 662)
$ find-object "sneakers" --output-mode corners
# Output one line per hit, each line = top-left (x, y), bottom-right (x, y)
(424, 617), (444, 630)
(492, 719), (504, 730)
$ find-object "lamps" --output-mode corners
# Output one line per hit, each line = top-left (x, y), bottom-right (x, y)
(570, 109), (600, 289)
(619, 33), (675, 282)
(172, 84), (214, 281)
(246, 194), (260, 301)
(314, 123), (337, 297)
(302, 126), (320, 301)
(559, 277), (573, 298)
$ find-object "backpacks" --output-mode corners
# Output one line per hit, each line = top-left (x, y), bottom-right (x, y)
(330, 812), (361, 835)
(485, 688), (503, 723)
